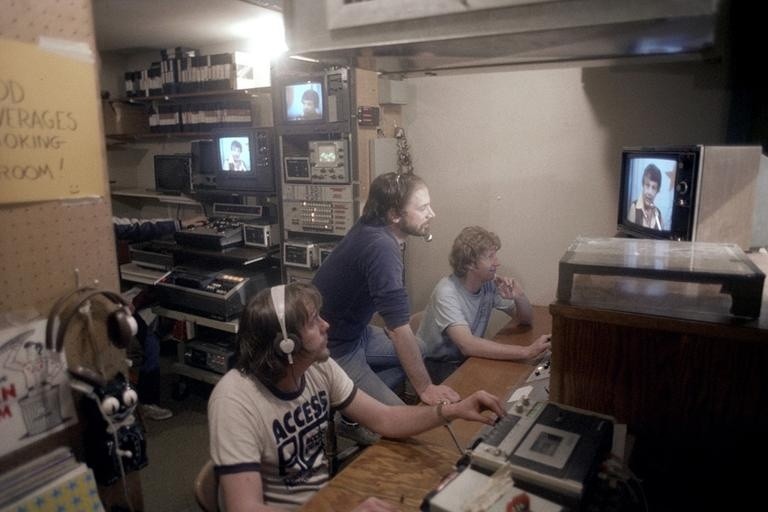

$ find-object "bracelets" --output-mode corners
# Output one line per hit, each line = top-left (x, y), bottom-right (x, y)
(437, 401), (452, 423)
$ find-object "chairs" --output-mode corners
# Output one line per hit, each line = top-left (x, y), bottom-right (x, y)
(400, 310), (425, 402)
(193, 458), (224, 512)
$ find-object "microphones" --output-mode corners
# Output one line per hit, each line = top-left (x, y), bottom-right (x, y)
(424, 230), (434, 244)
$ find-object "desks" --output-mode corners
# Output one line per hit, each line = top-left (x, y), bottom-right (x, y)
(297, 304), (556, 512)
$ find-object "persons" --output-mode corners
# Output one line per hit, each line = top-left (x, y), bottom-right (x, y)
(296, 90), (322, 120)
(309, 173), (462, 444)
(3, 342), (73, 441)
(208, 282), (508, 512)
(113, 214), (209, 422)
(415, 226), (552, 385)
(223, 142), (247, 172)
(628, 164), (664, 231)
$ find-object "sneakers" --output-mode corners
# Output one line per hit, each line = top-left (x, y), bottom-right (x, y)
(139, 404), (173, 420)
(337, 419), (379, 445)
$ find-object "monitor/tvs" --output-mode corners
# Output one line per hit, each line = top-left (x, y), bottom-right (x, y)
(616, 144), (767, 253)
(278, 69), (351, 126)
(154, 154), (194, 197)
(212, 126), (278, 192)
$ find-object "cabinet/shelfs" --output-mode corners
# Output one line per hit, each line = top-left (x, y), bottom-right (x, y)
(269, 63), (404, 282)
(128, 235), (281, 399)
(105, 89), (258, 208)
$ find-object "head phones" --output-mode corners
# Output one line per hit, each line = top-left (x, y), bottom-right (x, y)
(47, 286), (138, 395)
(270, 285), (301, 365)
